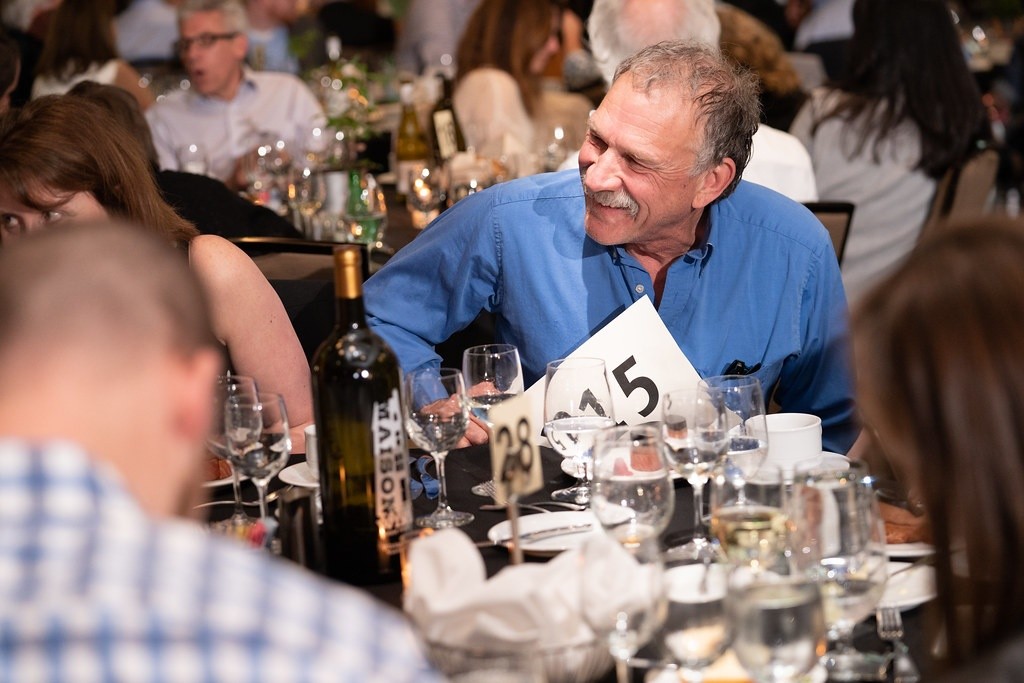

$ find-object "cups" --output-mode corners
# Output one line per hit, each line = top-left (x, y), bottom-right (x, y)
(743, 412), (823, 482)
(303, 425), (320, 479)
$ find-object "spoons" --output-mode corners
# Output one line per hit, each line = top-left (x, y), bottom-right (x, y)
(192, 484), (294, 512)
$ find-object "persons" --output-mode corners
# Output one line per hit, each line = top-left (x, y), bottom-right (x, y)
(0, 0), (1024, 313)
(0, 93), (315, 481)
(0, 216), (446, 683)
(850, 220), (1024, 683)
(361, 38), (863, 456)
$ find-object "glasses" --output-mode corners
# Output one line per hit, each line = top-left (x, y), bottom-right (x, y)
(174, 32), (237, 51)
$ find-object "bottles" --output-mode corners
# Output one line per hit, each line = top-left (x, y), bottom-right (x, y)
(392, 74), (438, 203)
(342, 170), (377, 247)
(315, 246), (407, 589)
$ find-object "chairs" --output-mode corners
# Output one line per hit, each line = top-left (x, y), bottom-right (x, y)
(802, 201), (854, 267)
(226, 236), (369, 363)
(918, 147), (1000, 241)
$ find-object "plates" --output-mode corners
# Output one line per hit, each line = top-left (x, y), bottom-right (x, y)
(863, 559), (938, 617)
(870, 538), (936, 561)
(736, 451), (849, 486)
(275, 460), (322, 488)
(485, 511), (624, 556)
(201, 466), (253, 490)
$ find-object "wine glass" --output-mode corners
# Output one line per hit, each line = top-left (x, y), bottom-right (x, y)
(176, 112), (582, 242)
(426, 100), (462, 161)
(130, 342), (890, 683)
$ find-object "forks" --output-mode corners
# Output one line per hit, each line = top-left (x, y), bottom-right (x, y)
(876, 608), (906, 683)
(479, 475), (550, 513)
